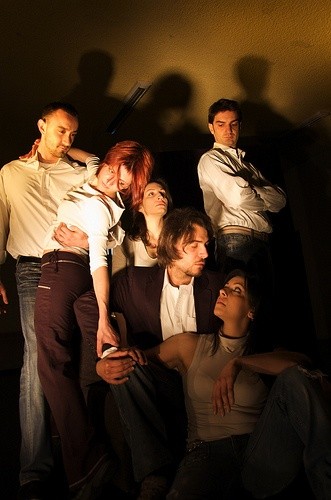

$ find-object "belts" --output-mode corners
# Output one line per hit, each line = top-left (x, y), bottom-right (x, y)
(16, 255), (42, 263)
(217, 225), (268, 242)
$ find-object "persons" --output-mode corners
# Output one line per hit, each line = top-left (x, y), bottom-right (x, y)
(19, 140), (155, 499)
(0, 102), (115, 500)
(73, 207), (230, 499)
(198, 98), (286, 331)
(122, 178), (183, 268)
(96, 269), (330, 500)
